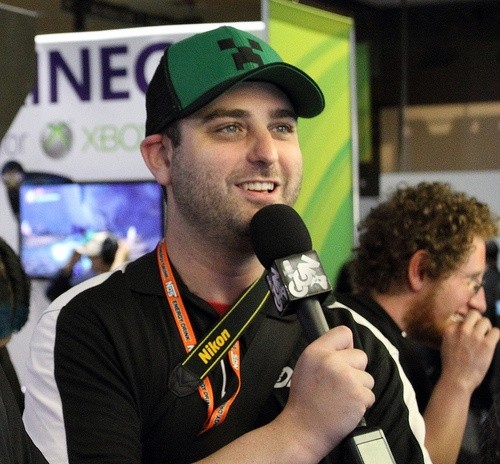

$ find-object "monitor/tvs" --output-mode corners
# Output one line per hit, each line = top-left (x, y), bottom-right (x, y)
(20, 179), (165, 279)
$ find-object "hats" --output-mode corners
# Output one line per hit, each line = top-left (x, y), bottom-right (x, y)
(75, 232), (112, 257)
(146, 26), (326, 135)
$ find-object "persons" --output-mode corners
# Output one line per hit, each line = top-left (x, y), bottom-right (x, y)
(0, 235), (32, 464)
(334, 182), (500, 464)
(23, 27), (431, 464)
(45, 229), (135, 303)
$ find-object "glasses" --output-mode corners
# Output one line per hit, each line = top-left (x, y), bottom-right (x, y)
(445, 259), (485, 296)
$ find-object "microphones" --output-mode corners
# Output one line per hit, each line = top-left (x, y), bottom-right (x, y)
(248, 204), (367, 430)
